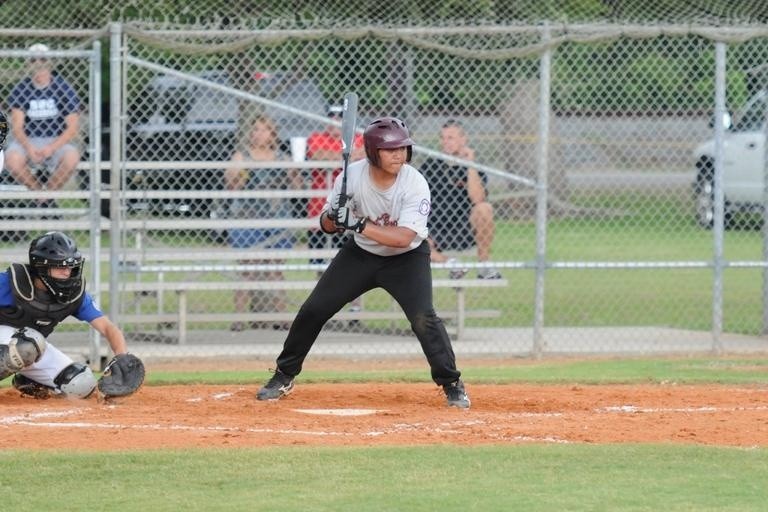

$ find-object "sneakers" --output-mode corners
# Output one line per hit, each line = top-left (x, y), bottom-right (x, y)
(12, 375), (50, 401)
(256, 372), (295, 401)
(449, 265), (502, 280)
(443, 376), (471, 410)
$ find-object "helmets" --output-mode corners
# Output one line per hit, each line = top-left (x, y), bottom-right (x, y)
(28, 231), (85, 307)
(363, 117), (414, 167)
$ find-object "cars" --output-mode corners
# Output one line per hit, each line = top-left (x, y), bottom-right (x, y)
(77, 70), (384, 223)
(693, 90), (768, 228)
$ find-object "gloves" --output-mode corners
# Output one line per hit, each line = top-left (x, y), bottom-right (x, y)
(326, 192), (355, 221)
(335, 206), (367, 234)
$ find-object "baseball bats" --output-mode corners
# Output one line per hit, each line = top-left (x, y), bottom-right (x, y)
(337, 93), (358, 233)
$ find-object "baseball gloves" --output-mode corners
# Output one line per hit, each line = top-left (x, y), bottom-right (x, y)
(97, 353), (144, 395)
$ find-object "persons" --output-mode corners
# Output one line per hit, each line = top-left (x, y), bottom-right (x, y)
(3, 42), (82, 222)
(0, 230), (129, 406)
(253, 118), (472, 411)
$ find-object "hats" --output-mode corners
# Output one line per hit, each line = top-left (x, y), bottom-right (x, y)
(28, 43), (48, 52)
